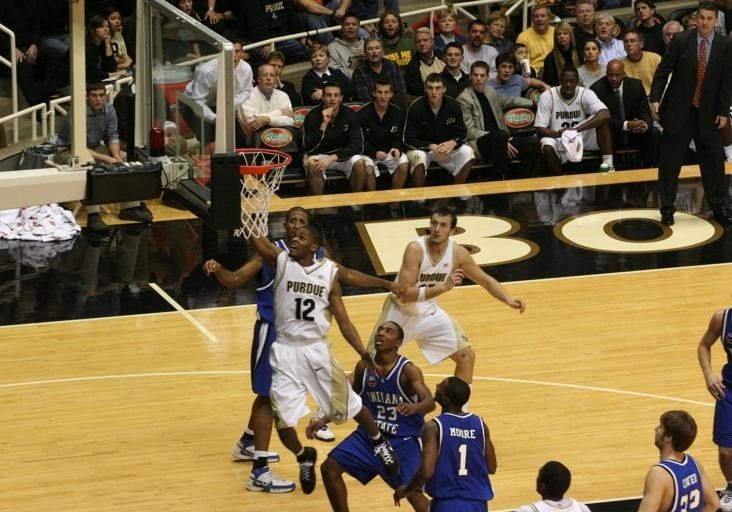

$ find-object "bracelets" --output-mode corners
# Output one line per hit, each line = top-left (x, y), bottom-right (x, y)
(416, 287), (428, 302)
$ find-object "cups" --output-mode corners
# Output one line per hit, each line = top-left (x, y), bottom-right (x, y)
(519, 59), (531, 76)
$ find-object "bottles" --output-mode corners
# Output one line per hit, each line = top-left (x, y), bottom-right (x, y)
(600, 162), (609, 172)
(149, 118), (165, 157)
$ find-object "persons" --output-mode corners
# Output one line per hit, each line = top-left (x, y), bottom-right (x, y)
(228, 213), (382, 493)
(698, 308), (732, 512)
(306, 322), (435, 512)
(396, 377), (497, 512)
(638, 410), (721, 512)
(505, 461), (591, 512)
(1, 0), (732, 229)
(203, 206), (408, 494)
(31, 228), (150, 318)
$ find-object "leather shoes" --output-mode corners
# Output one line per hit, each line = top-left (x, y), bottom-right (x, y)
(714, 210), (732, 225)
(87, 213), (108, 230)
(661, 212), (675, 225)
(118, 205), (153, 221)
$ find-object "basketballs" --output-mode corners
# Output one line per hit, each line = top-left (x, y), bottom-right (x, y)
(201, 141), (216, 155)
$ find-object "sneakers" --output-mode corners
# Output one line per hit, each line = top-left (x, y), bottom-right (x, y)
(719, 490), (732, 512)
(374, 436), (400, 478)
(296, 447), (317, 495)
(310, 418), (334, 441)
(600, 163), (615, 173)
(246, 466), (296, 493)
(232, 430), (281, 463)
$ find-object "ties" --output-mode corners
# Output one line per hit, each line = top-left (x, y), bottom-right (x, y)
(617, 90), (625, 120)
(693, 39), (706, 109)
